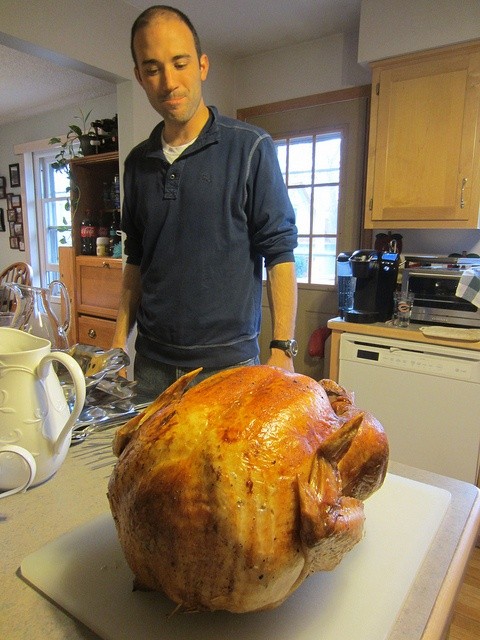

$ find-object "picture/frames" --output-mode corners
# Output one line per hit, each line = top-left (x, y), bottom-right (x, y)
(5, 193), (26, 252)
(0, 208), (5, 232)
(9, 163), (21, 187)
(0, 178), (5, 198)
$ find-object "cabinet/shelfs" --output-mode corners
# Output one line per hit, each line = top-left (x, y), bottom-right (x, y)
(362, 39), (480, 229)
(68, 154), (124, 346)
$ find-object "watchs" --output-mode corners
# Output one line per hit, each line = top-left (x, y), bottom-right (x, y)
(270, 338), (298, 358)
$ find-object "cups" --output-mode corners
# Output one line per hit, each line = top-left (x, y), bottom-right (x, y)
(396, 291), (416, 327)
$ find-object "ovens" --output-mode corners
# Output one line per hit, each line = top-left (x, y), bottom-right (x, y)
(398, 255), (480, 327)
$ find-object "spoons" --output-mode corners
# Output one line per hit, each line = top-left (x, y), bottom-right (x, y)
(71, 411), (141, 444)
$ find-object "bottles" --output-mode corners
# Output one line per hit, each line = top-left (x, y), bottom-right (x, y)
(113, 176), (120, 210)
(109, 225), (116, 255)
(80, 207), (97, 255)
(96, 209), (108, 236)
(98, 168), (114, 211)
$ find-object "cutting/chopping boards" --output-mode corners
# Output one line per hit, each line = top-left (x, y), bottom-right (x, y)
(20, 470), (452, 640)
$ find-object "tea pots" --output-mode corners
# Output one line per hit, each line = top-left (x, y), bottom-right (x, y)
(0, 281), (71, 375)
(0, 327), (86, 491)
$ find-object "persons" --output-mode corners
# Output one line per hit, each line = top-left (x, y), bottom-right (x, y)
(109, 3), (299, 405)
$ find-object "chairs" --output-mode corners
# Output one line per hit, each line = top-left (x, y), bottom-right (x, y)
(0, 261), (36, 337)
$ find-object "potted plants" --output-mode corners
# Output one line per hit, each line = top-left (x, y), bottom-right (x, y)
(48, 104), (98, 247)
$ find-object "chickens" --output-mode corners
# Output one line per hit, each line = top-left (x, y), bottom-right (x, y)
(108, 365), (388, 615)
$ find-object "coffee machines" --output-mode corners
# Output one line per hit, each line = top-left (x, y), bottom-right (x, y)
(336, 249), (394, 323)
